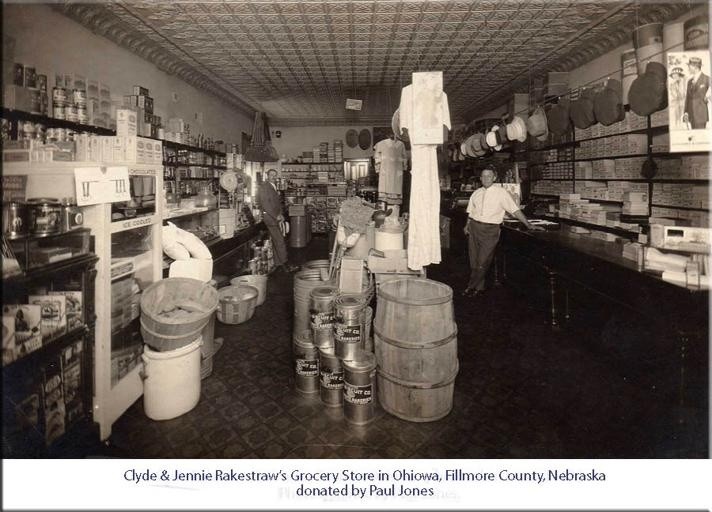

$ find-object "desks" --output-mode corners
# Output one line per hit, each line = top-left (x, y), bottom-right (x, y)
(494, 217), (709, 431)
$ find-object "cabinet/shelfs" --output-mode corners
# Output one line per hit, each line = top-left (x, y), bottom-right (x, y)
(0, 108), (355, 459)
(527, 106), (710, 239)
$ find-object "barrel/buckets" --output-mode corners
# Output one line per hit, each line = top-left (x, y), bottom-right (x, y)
(319, 343), (342, 405)
(292, 270), (336, 348)
(295, 347), (320, 395)
(374, 280), (459, 423)
(139, 340), (207, 421)
(342, 349), (377, 425)
(310, 287), (333, 346)
(333, 293), (366, 357)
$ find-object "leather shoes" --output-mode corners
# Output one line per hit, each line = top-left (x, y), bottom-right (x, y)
(457, 285), (486, 299)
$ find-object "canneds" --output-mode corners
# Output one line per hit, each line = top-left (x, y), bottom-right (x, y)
(6, 60), (89, 237)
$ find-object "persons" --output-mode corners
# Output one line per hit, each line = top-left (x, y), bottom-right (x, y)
(668, 67), (689, 130)
(439, 179), (456, 250)
(257, 169), (300, 273)
(462, 163), (544, 297)
(682, 57), (711, 129)
